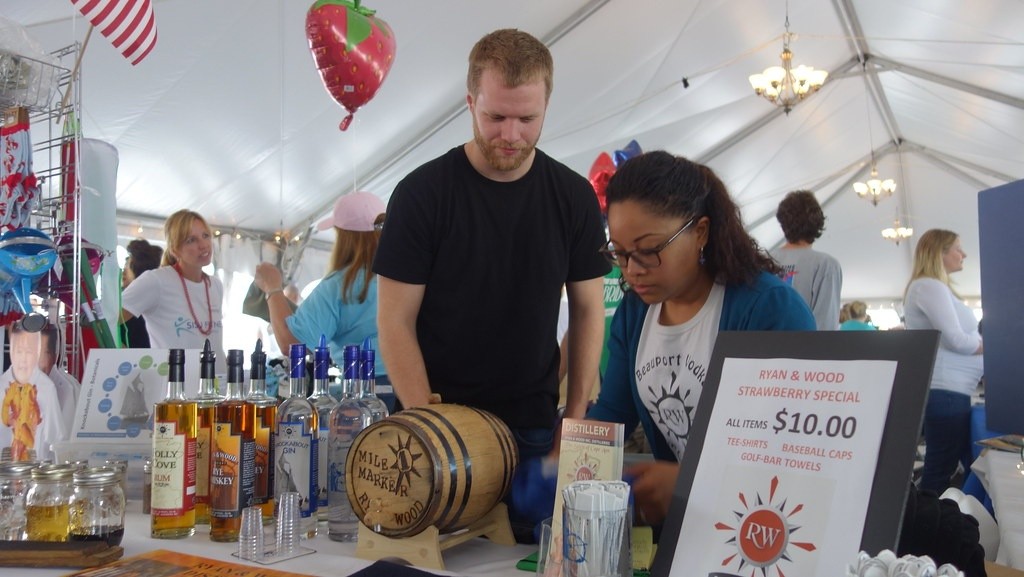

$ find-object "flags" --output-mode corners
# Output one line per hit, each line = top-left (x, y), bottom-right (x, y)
(70, 0), (158, 64)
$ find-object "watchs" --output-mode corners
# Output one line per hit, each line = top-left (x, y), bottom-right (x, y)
(265, 287), (283, 302)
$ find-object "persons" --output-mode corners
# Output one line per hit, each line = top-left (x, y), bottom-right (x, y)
(901, 229), (1024, 577)
(0, 318), (81, 465)
(120, 209), (228, 396)
(578, 150), (815, 509)
(763, 190), (878, 331)
(254, 191), (399, 413)
(372, 28), (613, 501)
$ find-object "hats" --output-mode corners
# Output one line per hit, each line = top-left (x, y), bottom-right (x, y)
(317, 191), (388, 232)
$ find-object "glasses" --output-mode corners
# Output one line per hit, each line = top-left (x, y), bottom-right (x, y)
(599, 216), (699, 268)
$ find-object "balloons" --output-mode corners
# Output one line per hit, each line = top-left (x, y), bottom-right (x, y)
(591, 139), (643, 219)
(306, 0), (398, 132)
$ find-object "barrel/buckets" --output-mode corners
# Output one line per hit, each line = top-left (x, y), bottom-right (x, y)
(345, 401), (523, 538)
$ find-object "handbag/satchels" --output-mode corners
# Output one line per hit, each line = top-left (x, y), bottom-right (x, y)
(242, 280), (297, 323)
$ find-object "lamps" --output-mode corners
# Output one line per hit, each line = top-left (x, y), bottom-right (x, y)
(880, 138), (913, 246)
(748, 1), (828, 115)
(850, 54), (897, 208)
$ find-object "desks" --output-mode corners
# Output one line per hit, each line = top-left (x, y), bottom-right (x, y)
(970, 447), (1024, 571)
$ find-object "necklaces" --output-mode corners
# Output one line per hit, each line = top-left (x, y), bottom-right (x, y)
(176, 263), (212, 335)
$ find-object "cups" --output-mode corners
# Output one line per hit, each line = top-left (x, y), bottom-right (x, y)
(274, 491), (300, 556)
(237, 508), (267, 558)
(561, 503), (634, 576)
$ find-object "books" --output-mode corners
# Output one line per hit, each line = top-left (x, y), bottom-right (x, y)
(541, 418), (626, 577)
(61, 548), (322, 577)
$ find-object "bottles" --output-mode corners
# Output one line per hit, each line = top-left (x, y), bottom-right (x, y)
(0, 446), (127, 552)
(148, 334), (390, 542)
